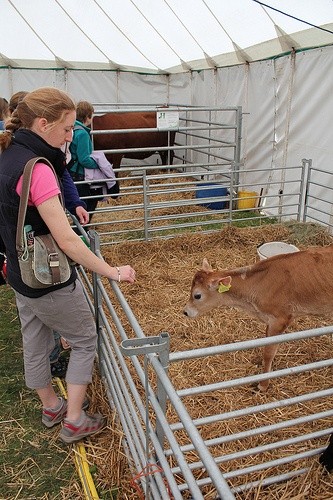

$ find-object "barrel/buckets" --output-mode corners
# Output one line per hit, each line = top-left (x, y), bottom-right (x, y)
(195, 182), (228, 210)
(257, 241), (301, 263)
(237, 191), (258, 211)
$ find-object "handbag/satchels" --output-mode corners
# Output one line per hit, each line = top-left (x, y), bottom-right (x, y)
(18, 157), (71, 289)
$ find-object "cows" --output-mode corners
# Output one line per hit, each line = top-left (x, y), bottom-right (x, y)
(185, 247), (333, 390)
(88, 110), (177, 172)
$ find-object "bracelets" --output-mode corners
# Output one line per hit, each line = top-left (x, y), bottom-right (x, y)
(115, 267), (121, 282)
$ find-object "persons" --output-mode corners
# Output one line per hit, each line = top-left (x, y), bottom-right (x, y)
(0, 87), (136, 444)
(0, 91), (89, 285)
(65, 101), (100, 235)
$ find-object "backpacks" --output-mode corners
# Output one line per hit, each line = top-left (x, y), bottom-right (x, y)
(61, 123), (85, 164)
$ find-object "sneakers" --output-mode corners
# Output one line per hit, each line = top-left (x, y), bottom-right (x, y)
(60, 414), (103, 443)
(48, 344), (65, 362)
(42, 394), (89, 427)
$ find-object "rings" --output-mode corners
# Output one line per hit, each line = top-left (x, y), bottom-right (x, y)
(132, 273), (134, 275)
